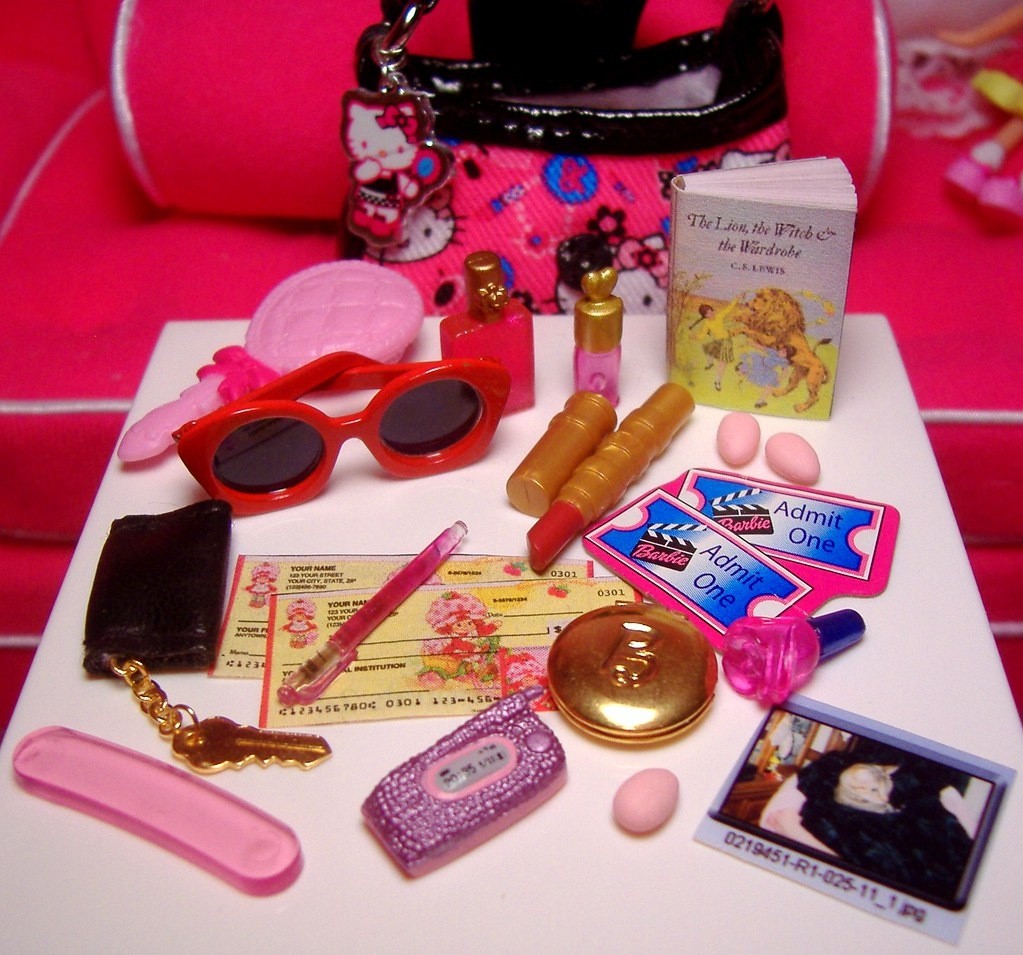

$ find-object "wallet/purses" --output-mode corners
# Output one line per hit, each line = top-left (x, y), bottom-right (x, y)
(80, 496), (234, 679)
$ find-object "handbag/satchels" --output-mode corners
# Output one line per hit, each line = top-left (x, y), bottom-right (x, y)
(339, 0), (793, 318)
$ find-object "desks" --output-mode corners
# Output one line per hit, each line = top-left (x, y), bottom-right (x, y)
(3, 323), (1023, 954)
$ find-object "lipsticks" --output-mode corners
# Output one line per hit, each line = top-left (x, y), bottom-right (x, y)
(526, 380), (699, 576)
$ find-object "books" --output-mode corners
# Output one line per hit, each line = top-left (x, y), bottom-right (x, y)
(663, 155), (860, 422)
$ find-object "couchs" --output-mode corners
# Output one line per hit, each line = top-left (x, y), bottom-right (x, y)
(0, 0), (1020, 717)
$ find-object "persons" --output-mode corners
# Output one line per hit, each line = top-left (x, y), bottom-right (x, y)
(933, 1), (1023, 171)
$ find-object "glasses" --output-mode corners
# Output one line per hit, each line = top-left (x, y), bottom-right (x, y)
(168, 349), (513, 517)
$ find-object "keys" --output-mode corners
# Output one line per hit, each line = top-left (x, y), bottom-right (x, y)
(175, 715), (332, 773)
